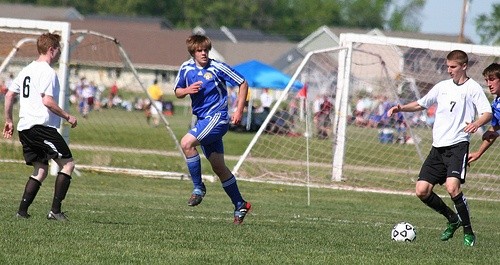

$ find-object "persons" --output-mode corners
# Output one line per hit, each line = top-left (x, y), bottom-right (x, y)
(388, 50), (492, 246)
(173, 35), (252, 223)
(468, 62), (500, 164)
(0, 76), (487, 147)
(3, 34), (77, 222)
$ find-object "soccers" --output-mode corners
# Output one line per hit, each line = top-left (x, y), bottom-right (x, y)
(390, 222), (416, 244)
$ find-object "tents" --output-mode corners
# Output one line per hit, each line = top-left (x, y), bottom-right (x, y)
(227, 59), (304, 130)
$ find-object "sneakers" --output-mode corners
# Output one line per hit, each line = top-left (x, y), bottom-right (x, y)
(47, 210), (70, 222)
(464, 232), (475, 247)
(440, 218), (461, 241)
(187, 183), (206, 207)
(232, 201), (251, 225)
(16, 212), (31, 221)
